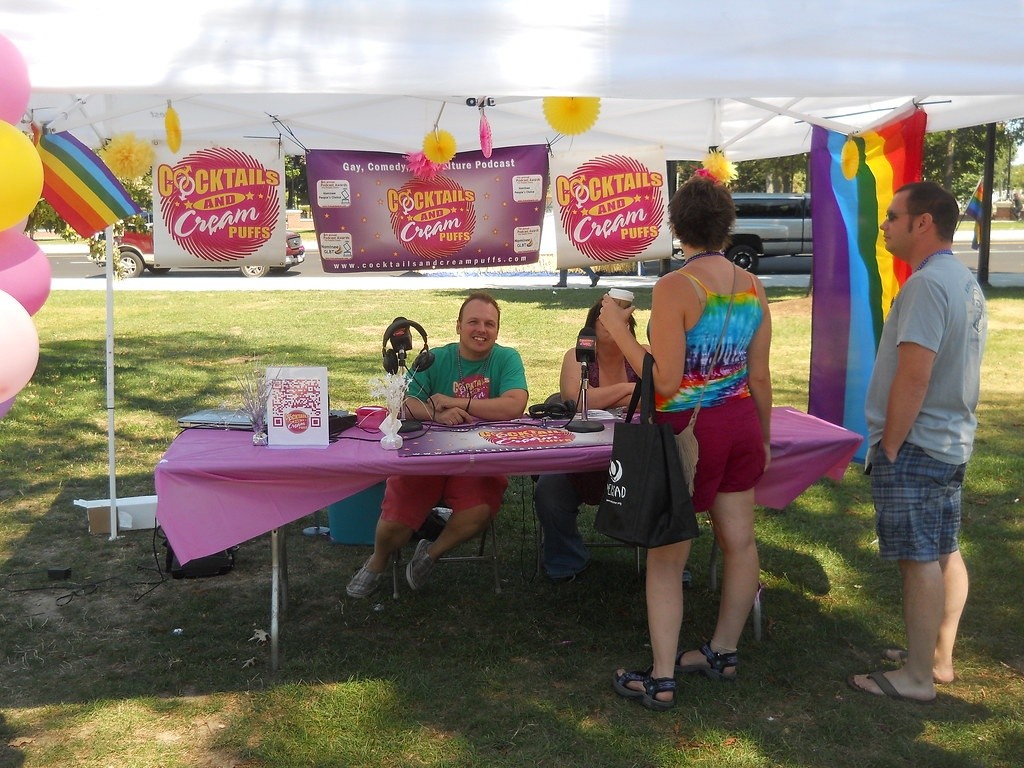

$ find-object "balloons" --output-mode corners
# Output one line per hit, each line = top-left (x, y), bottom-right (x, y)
(0, 36), (51, 421)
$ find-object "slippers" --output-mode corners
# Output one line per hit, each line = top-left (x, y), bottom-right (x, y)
(847, 671), (938, 704)
(883, 648), (957, 684)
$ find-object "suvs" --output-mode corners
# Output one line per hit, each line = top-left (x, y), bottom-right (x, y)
(728, 194), (812, 274)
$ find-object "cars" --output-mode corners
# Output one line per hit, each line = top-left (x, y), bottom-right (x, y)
(90, 211), (306, 278)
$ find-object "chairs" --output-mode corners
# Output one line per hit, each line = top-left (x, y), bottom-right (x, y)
(530, 392), (640, 577)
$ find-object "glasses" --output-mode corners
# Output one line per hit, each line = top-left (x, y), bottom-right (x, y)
(886, 211), (936, 224)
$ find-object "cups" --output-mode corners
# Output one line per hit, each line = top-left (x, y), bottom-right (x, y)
(607, 288), (634, 309)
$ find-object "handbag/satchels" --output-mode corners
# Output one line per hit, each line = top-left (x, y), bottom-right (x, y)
(593, 352), (702, 548)
(671, 427), (699, 497)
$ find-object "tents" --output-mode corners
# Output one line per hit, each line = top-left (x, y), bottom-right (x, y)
(0, 0), (1024, 168)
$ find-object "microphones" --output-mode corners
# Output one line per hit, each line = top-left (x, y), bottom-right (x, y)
(389, 317), (412, 365)
(575, 327), (597, 379)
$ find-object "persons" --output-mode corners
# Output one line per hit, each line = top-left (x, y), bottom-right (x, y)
(552, 266), (599, 287)
(847, 182), (987, 705)
(598, 180), (773, 710)
(1009, 194), (1022, 221)
(535, 293), (692, 584)
(346, 293), (528, 598)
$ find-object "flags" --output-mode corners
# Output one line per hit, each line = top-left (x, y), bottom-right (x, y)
(34, 131), (142, 238)
(966, 177), (984, 249)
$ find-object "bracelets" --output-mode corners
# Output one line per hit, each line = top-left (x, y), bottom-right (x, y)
(465, 398), (472, 413)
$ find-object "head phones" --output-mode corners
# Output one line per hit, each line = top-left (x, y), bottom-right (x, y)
(529, 399), (576, 420)
(381, 320), (435, 375)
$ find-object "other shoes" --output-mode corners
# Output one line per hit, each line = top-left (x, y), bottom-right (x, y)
(407, 538), (435, 590)
(346, 554), (390, 598)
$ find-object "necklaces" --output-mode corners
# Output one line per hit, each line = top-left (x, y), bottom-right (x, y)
(682, 249), (727, 268)
(457, 346), (492, 399)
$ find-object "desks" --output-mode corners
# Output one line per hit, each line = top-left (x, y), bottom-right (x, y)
(153, 407), (861, 675)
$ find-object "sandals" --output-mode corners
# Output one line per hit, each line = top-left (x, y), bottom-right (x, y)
(674, 643), (737, 681)
(612, 666), (676, 711)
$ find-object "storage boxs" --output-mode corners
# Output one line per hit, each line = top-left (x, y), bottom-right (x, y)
(74, 495), (159, 533)
(328, 482), (387, 545)
